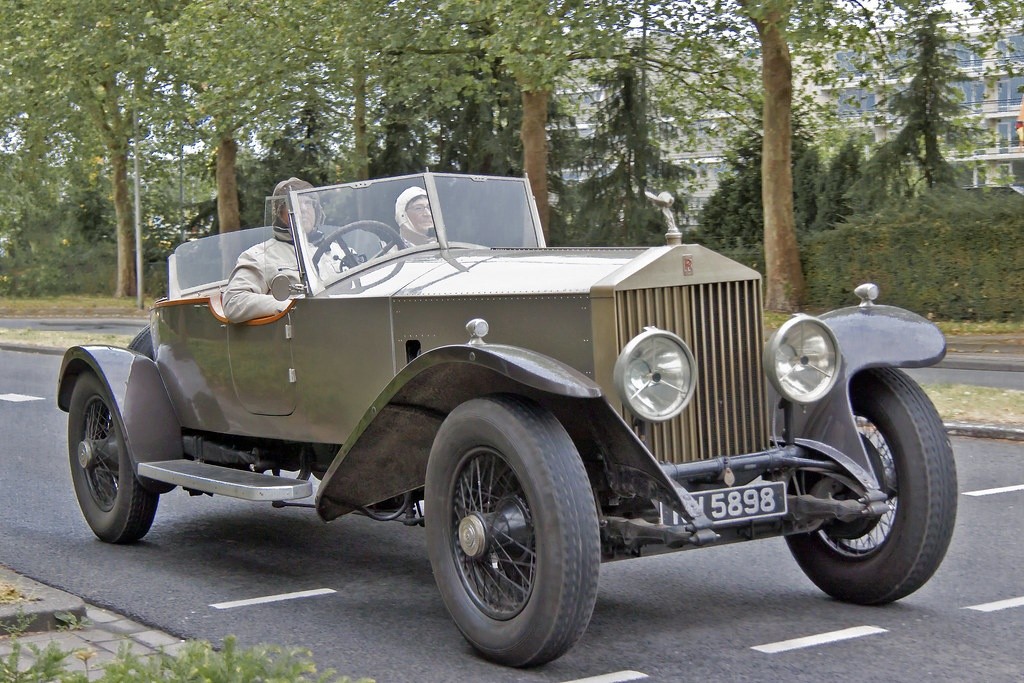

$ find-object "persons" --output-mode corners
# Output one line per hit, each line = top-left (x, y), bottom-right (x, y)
(382, 186), (438, 253)
(221, 177), (358, 323)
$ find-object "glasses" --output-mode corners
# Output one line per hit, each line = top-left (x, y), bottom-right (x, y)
(405, 203), (431, 214)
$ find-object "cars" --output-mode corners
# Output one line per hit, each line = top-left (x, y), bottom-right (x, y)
(56, 168), (961, 672)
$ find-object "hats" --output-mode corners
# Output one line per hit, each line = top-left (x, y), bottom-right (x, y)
(272, 177), (324, 241)
(395, 186), (434, 244)
